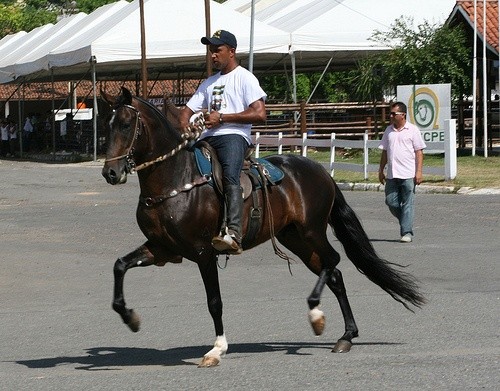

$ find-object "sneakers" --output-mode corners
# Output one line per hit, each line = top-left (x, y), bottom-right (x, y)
(400, 233), (412, 242)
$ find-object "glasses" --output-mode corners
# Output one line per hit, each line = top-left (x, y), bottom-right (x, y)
(389, 112), (407, 117)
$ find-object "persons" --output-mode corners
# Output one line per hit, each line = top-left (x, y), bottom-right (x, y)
(176, 30), (268, 255)
(377, 101), (428, 243)
(0, 110), (76, 159)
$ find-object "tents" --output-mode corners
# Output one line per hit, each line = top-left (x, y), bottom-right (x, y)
(219, 0), (461, 155)
(49, 0), (299, 163)
(0, 0), (133, 156)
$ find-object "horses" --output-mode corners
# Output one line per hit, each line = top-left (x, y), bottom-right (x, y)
(99, 81), (427, 371)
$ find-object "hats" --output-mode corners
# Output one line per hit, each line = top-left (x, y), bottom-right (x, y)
(201, 30), (237, 49)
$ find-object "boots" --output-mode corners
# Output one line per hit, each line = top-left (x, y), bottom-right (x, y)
(211, 184), (243, 255)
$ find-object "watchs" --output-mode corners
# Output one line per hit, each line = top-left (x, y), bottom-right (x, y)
(218, 113), (223, 124)
